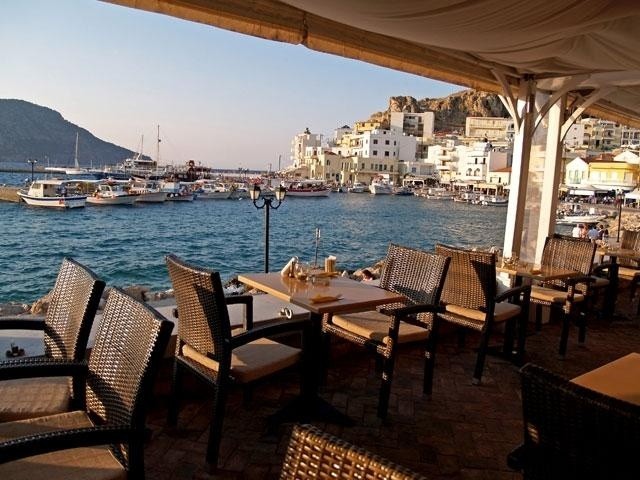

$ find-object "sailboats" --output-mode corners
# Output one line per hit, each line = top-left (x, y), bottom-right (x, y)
(44, 124), (222, 181)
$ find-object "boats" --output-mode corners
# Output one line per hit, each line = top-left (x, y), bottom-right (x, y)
(556, 202), (608, 226)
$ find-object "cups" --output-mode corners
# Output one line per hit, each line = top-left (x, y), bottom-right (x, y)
(294, 262), (309, 280)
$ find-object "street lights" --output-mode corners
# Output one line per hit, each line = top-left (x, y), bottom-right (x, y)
(250, 184), (286, 272)
(27, 159), (38, 181)
(616, 189), (624, 242)
(240, 168), (247, 180)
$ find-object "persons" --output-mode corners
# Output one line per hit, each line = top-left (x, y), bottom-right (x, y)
(573, 222), (610, 244)
(361, 270), (374, 281)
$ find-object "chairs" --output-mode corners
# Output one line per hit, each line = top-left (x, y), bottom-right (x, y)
(278, 422), (428, 480)
(521, 233), (597, 351)
(164, 252), (316, 474)
(434, 242), (531, 385)
(596, 230), (640, 295)
(322, 244), (451, 417)
(518, 352), (640, 480)
(0, 256), (106, 420)
(0, 286), (174, 480)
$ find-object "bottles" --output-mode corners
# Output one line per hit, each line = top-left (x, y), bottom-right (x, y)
(324, 258), (336, 273)
